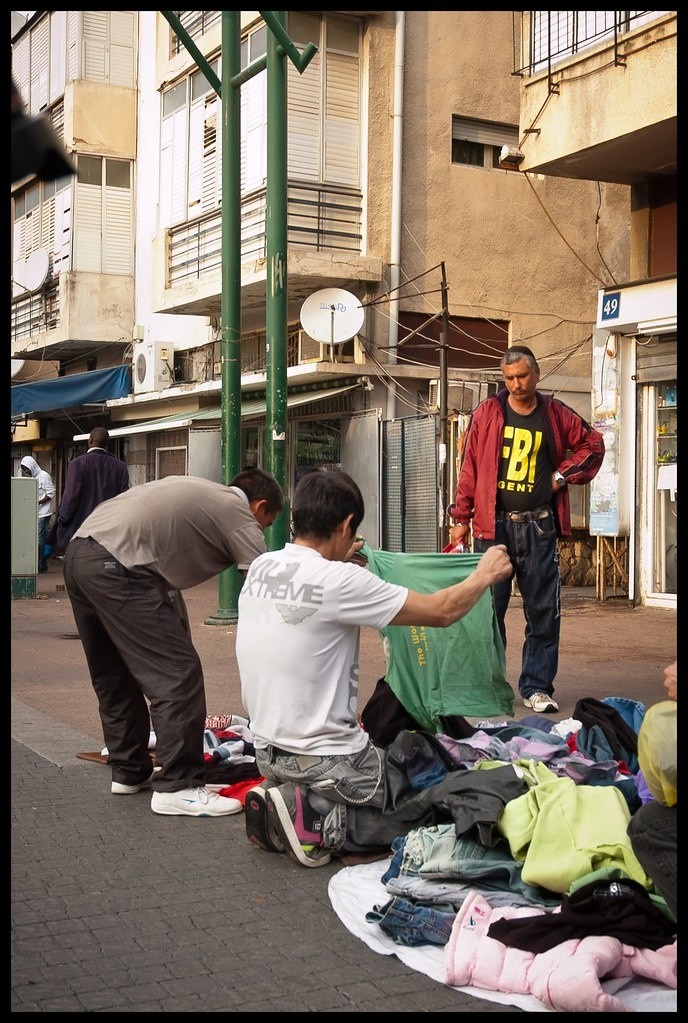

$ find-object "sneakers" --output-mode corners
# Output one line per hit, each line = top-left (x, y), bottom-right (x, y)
(523, 690), (558, 712)
(244, 781), (285, 854)
(110, 767), (159, 793)
(265, 779), (331, 867)
(151, 787), (242, 817)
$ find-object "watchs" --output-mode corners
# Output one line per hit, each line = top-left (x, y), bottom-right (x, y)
(554, 471), (565, 486)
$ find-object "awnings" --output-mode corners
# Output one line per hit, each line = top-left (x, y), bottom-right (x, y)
(11, 363), (132, 427)
(72, 385), (361, 440)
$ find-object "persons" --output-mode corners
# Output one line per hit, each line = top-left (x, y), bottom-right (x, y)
(62, 465), (284, 817)
(627, 659), (676, 921)
(446, 345), (607, 712)
(20, 457), (57, 573)
(234, 467), (513, 867)
(43, 427), (130, 550)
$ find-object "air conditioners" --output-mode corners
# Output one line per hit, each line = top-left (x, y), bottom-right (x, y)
(132, 340), (174, 394)
(429, 379), (488, 422)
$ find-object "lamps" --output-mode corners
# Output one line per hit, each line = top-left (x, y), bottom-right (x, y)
(499, 144), (526, 167)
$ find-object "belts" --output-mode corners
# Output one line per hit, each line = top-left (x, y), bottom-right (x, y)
(495, 506), (553, 522)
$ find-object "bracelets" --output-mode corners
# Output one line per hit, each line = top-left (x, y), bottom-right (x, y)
(456, 523), (463, 526)
